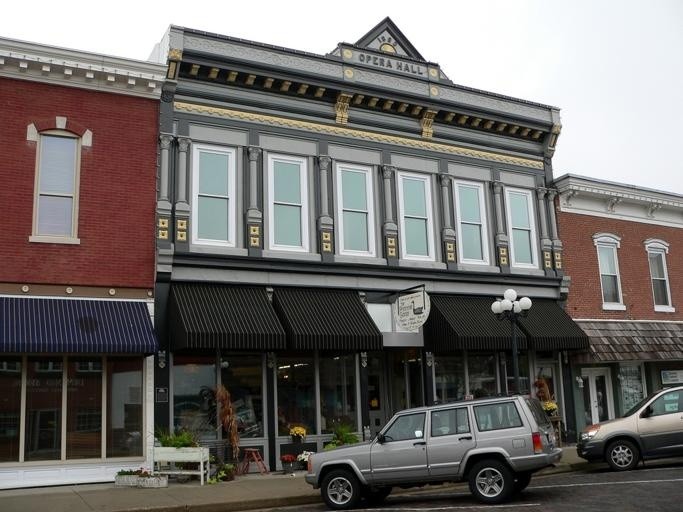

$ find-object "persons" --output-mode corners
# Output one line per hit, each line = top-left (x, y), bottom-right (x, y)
(278, 407), (286, 426)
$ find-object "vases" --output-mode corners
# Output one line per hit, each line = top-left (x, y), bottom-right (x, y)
(282, 461), (292, 473)
(292, 435), (301, 444)
(545, 410), (554, 416)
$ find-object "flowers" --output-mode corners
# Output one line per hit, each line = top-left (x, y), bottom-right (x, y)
(542, 402), (558, 410)
(289, 425), (306, 440)
(279, 450), (315, 463)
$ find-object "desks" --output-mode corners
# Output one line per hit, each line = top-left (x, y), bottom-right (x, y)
(153, 446), (210, 485)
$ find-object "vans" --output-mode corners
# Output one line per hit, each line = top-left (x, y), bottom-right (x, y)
(457, 377), (529, 400)
(577, 387), (683, 470)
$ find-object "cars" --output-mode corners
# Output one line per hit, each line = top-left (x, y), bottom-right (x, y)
(172, 397), (259, 439)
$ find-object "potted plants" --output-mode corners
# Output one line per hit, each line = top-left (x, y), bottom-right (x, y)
(113, 468), (169, 488)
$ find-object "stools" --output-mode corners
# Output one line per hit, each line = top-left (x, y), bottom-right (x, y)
(242, 447), (268, 475)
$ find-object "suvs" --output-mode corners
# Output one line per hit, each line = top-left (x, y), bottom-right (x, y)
(304, 394), (564, 511)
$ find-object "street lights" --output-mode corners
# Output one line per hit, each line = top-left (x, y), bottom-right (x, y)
(492, 289), (533, 397)
